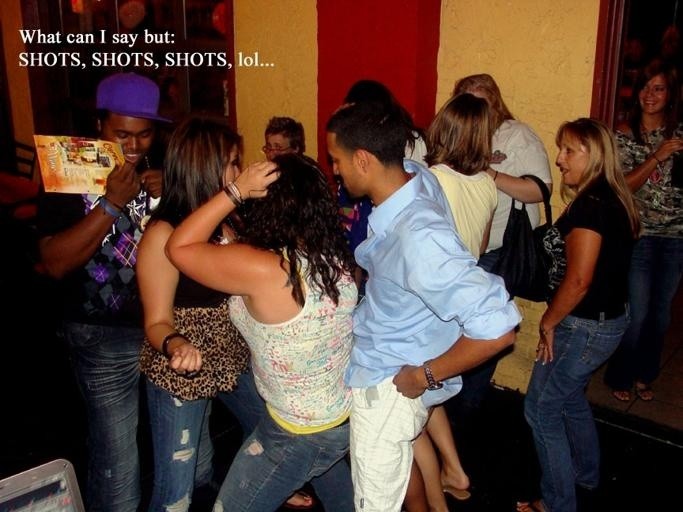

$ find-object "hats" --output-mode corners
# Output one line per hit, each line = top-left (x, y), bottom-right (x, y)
(96, 71), (176, 125)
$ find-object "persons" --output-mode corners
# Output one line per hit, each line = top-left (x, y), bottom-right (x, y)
(0, 24), (683, 512)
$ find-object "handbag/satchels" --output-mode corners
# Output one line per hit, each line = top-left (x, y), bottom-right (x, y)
(502, 174), (553, 303)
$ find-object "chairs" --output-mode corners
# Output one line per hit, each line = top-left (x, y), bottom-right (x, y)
(4, 136), (38, 183)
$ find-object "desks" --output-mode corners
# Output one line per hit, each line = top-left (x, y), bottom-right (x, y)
(0, 173), (39, 207)
